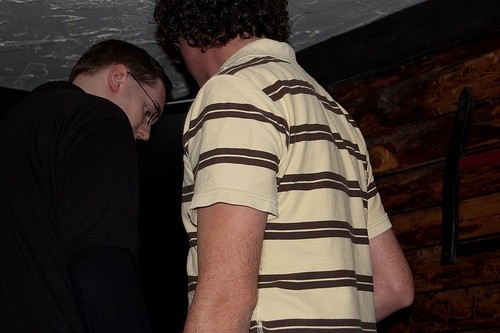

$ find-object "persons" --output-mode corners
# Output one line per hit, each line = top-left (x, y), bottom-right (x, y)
(1, 38), (188, 333)
(154, 0), (416, 333)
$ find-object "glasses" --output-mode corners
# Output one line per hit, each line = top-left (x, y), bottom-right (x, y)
(127, 72), (161, 127)
